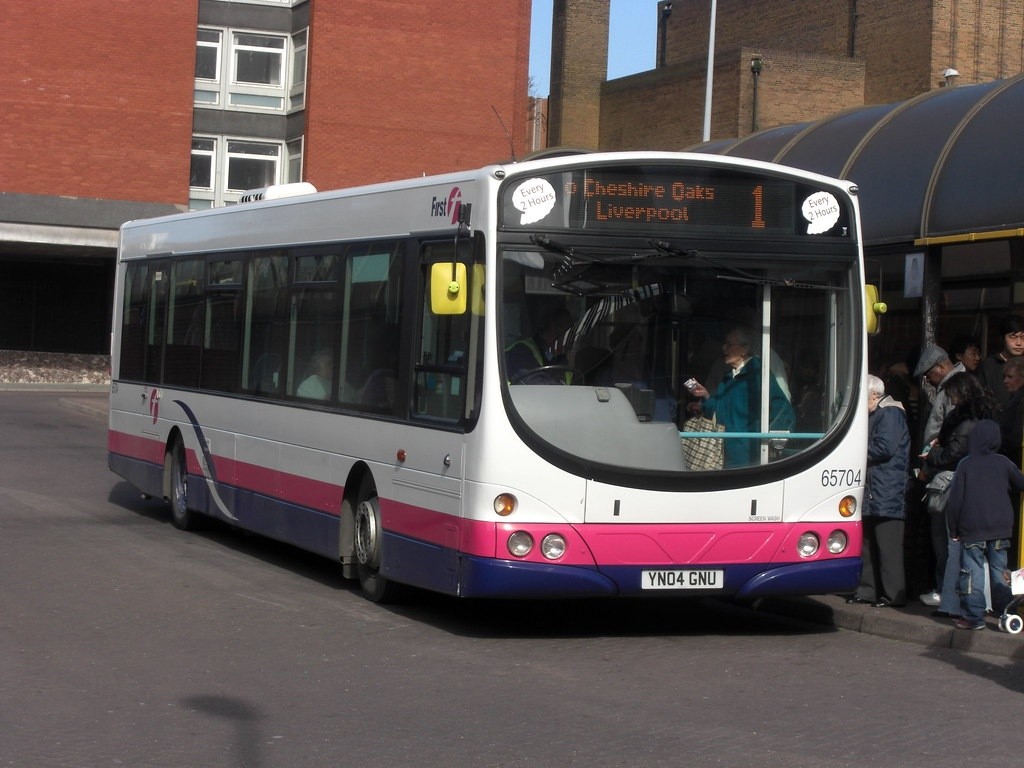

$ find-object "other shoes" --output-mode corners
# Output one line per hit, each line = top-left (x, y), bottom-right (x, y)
(931, 611), (962, 619)
(954, 618), (986, 630)
(920, 592), (941, 605)
(992, 608), (1016, 616)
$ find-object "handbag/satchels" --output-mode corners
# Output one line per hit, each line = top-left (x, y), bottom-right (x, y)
(682, 410), (726, 471)
(921, 470), (957, 516)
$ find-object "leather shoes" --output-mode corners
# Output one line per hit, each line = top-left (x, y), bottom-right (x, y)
(872, 599), (889, 607)
(847, 597), (866, 603)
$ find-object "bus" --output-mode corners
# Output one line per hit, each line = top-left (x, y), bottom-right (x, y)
(105, 146), (890, 607)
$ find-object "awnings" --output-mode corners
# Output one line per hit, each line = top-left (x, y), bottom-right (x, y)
(680, 72), (1024, 244)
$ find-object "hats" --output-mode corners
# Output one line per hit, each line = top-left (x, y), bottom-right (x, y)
(914, 345), (948, 375)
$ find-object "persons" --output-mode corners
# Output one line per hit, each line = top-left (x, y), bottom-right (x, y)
(504, 321), (585, 386)
(685, 317), (1024, 628)
(295, 349), (359, 404)
